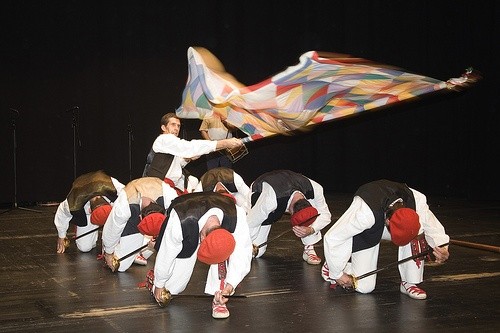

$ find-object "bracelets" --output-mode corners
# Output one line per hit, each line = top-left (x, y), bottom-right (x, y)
(311, 227), (313, 235)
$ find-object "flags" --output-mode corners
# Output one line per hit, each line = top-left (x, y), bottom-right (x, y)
(175, 46), (455, 142)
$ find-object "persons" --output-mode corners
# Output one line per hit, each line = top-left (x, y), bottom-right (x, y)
(152, 191), (253, 319)
(320, 180), (450, 300)
(52, 171), (125, 254)
(102, 176), (178, 273)
(248, 169), (332, 265)
(141, 113), (243, 195)
(193, 166), (251, 215)
(199, 112), (239, 171)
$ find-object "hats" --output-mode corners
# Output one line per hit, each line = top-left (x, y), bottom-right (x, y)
(137, 213), (167, 235)
(197, 229), (236, 264)
(389, 207), (420, 246)
(90, 204), (112, 225)
(291, 206), (318, 226)
(220, 192), (237, 203)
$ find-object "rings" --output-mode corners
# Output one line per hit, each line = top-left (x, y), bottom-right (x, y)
(343, 286), (346, 289)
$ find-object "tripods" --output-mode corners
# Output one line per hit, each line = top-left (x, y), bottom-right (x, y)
(0, 112), (44, 215)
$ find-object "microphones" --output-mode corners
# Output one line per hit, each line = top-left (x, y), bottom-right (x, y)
(64, 106), (77, 111)
(8, 107), (18, 113)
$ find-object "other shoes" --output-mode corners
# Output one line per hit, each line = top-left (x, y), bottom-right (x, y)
(303, 248), (322, 264)
(400, 281), (427, 299)
(320, 260), (330, 281)
(134, 254), (147, 265)
(211, 296), (230, 318)
